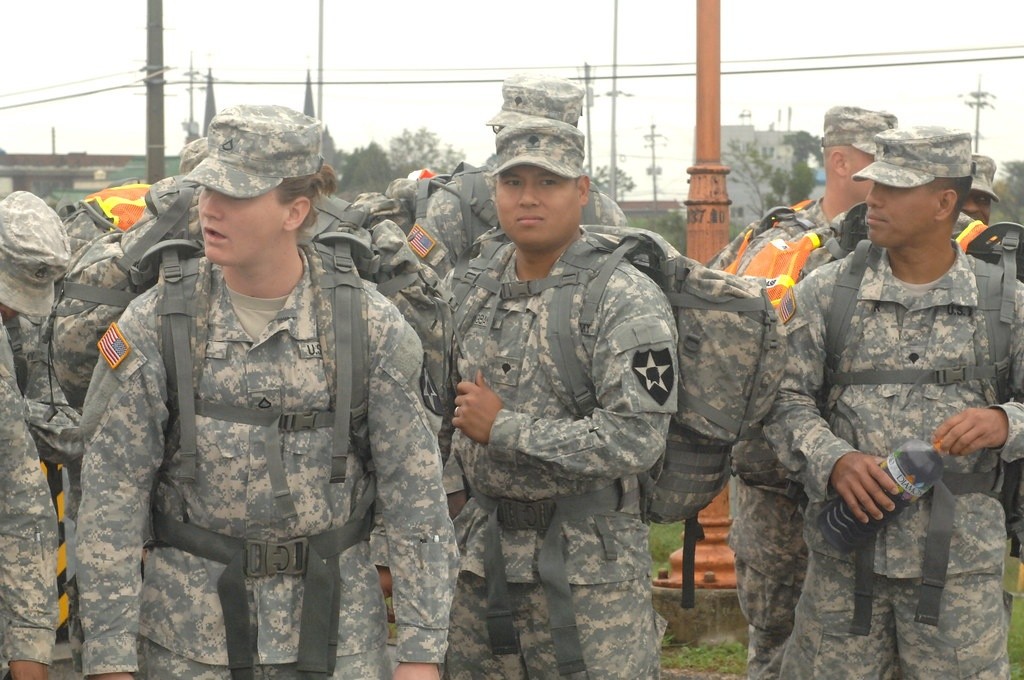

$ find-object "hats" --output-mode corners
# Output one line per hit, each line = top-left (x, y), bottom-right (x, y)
(822, 106), (898, 155)
(970, 154), (1000, 203)
(179, 138), (209, 177)
(486, 74), (585, 128)
(182, 105), (321, 200)
(851, 126), (976, 189)
(492, 117), (585, 178)
(0, 191), (71, 316)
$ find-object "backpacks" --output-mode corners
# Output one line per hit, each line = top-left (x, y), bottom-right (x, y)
(447, 223), (778, 526)
(705, 205), (1024, 530)
(48, 161), (493, 578)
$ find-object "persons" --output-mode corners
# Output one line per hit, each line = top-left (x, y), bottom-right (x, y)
(0, 71), (1023, 680)
(76, 103), (460, 680)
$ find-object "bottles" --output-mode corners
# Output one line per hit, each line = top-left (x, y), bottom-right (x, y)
(818, 437), (949, 556)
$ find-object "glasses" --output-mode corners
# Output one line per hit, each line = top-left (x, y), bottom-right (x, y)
(492, 125), (505, 134)
